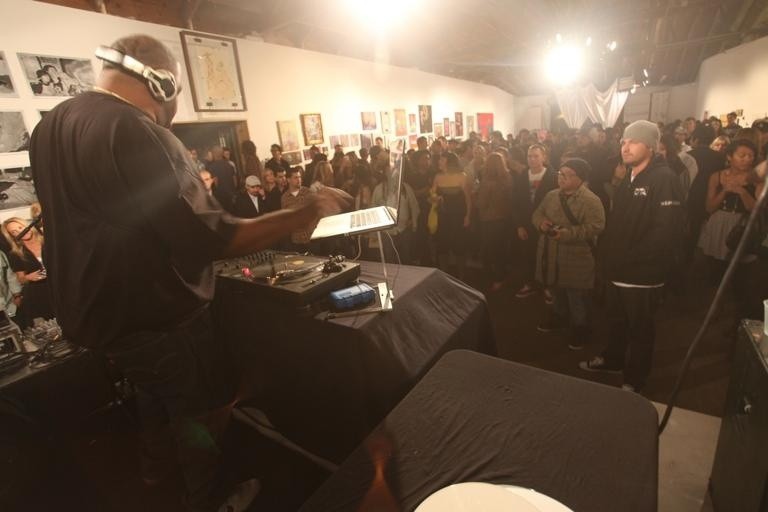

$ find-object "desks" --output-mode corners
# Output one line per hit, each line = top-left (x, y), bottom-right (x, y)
(300, 349), (658, 512)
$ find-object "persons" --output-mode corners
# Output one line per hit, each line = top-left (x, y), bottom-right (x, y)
(44, 65), (74, 96)
(0, 249), (26, 319)
(189, 112), (767, 400)
(36, 70), (54, 95)
(0, 218), (50, 324)
(29, 34), (356, 510)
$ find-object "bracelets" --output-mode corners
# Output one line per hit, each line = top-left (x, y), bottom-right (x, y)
(15, 295), (23, 299)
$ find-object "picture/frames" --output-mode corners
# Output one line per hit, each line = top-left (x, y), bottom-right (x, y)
(301, 113), (324, 145)
(179, 30), (247, 112)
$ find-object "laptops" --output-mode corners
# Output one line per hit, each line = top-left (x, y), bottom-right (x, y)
(310, 138), (405, 243)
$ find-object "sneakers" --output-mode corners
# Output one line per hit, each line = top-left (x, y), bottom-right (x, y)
(620, 384), (644, 394)
(536, 312), (569, 332)
(515, 284), (537, 298)
(578, 355), (624, 376)
(569, 322), (587, 349)
(543, 287), (557, 305)
(217, 477), (263, 512)
(486, 279), (507, 294)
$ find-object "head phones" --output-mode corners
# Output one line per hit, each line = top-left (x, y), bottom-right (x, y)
(95, 45), (177, 102)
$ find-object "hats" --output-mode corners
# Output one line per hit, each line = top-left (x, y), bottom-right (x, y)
(558, 158), (594, 181)
(622, 120), (660, 152)
(246, 175), (261, 187)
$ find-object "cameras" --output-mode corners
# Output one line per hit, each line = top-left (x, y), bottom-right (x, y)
(548, 226), (557, 236)
